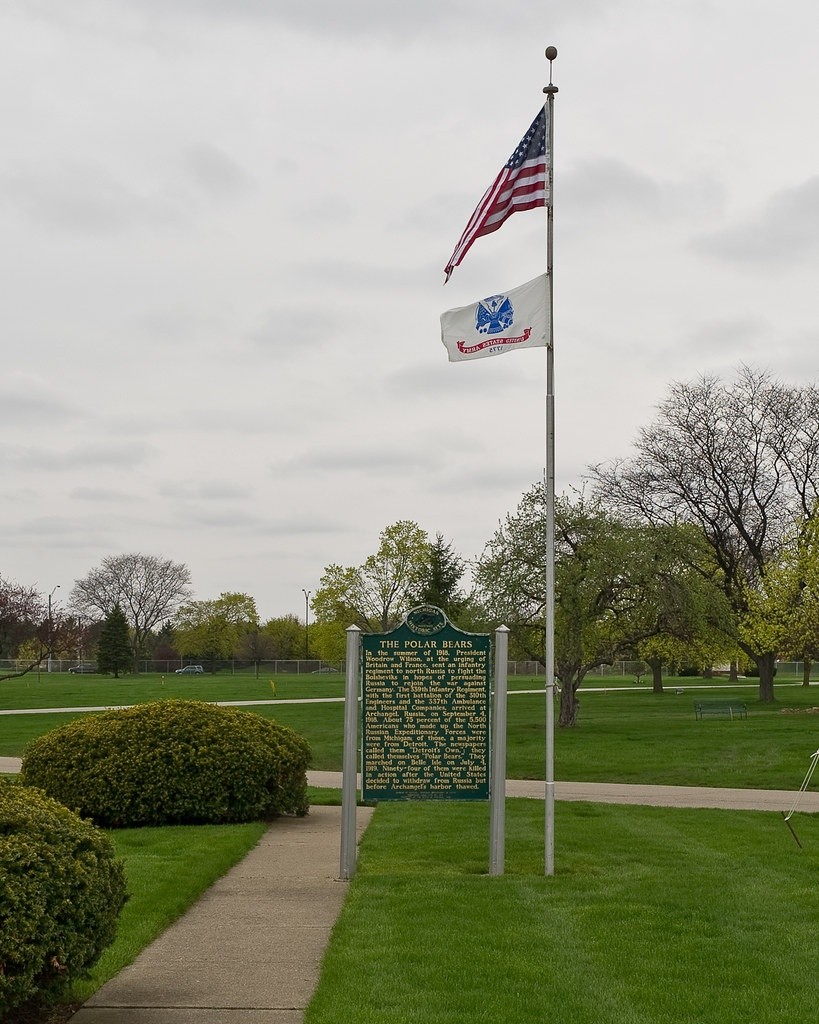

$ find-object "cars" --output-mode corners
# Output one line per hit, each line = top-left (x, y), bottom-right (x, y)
(176, 665), (204, 675)
(69, 663), (96, 675)
(312, 668), (338, 673)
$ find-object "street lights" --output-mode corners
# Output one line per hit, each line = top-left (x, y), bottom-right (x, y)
(302, 588), (311, 659)
(48, 585), (61, 632)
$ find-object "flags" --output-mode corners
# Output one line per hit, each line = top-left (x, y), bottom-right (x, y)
(443, 99), (552, 287)
(440, 275), (551, 362)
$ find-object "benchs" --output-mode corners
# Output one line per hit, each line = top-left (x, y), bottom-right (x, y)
(694, 702), (747, 721)
